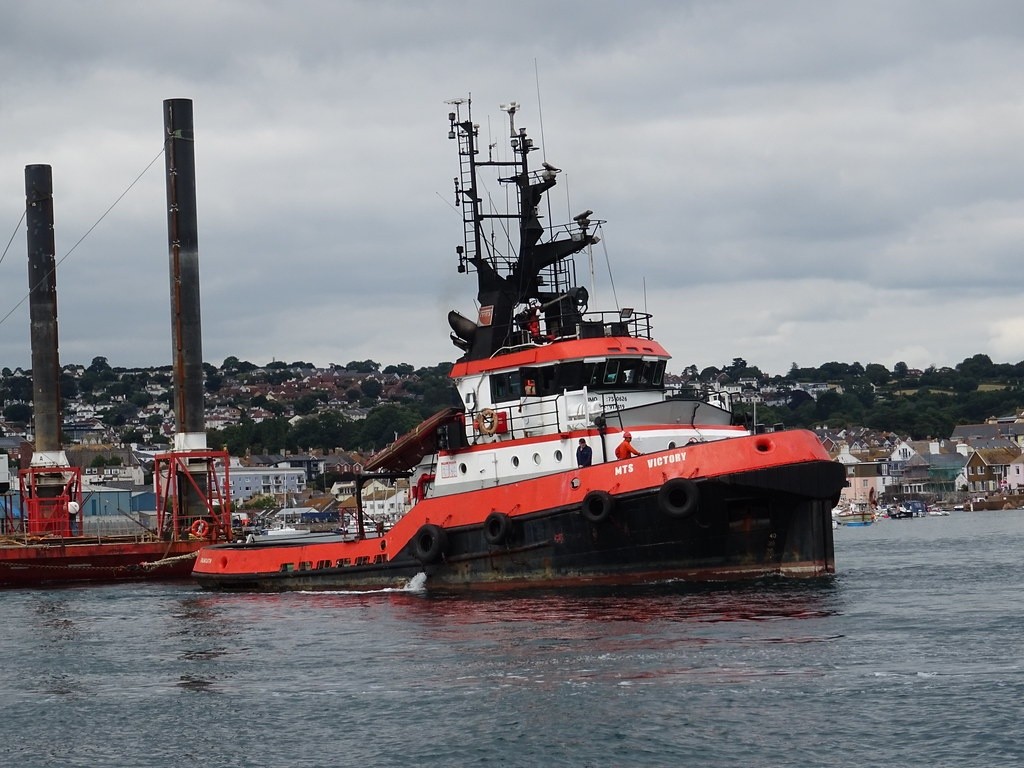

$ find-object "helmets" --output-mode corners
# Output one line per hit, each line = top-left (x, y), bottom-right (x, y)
(624, 432), (633, 437)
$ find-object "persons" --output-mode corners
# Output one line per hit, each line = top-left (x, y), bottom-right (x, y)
(577, 438), (593, 468)
(614, 432), (642, 460)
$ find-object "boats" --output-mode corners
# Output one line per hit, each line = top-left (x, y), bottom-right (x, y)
(189, 50), (839, 594)
(2, 96), (255, 587)
(831, 489), (1020, 528)
(229, 507), (406, 545)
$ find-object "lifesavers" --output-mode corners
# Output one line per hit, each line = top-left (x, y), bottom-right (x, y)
(191, 520), (208, 537)
(479, 409), (498, 435)
